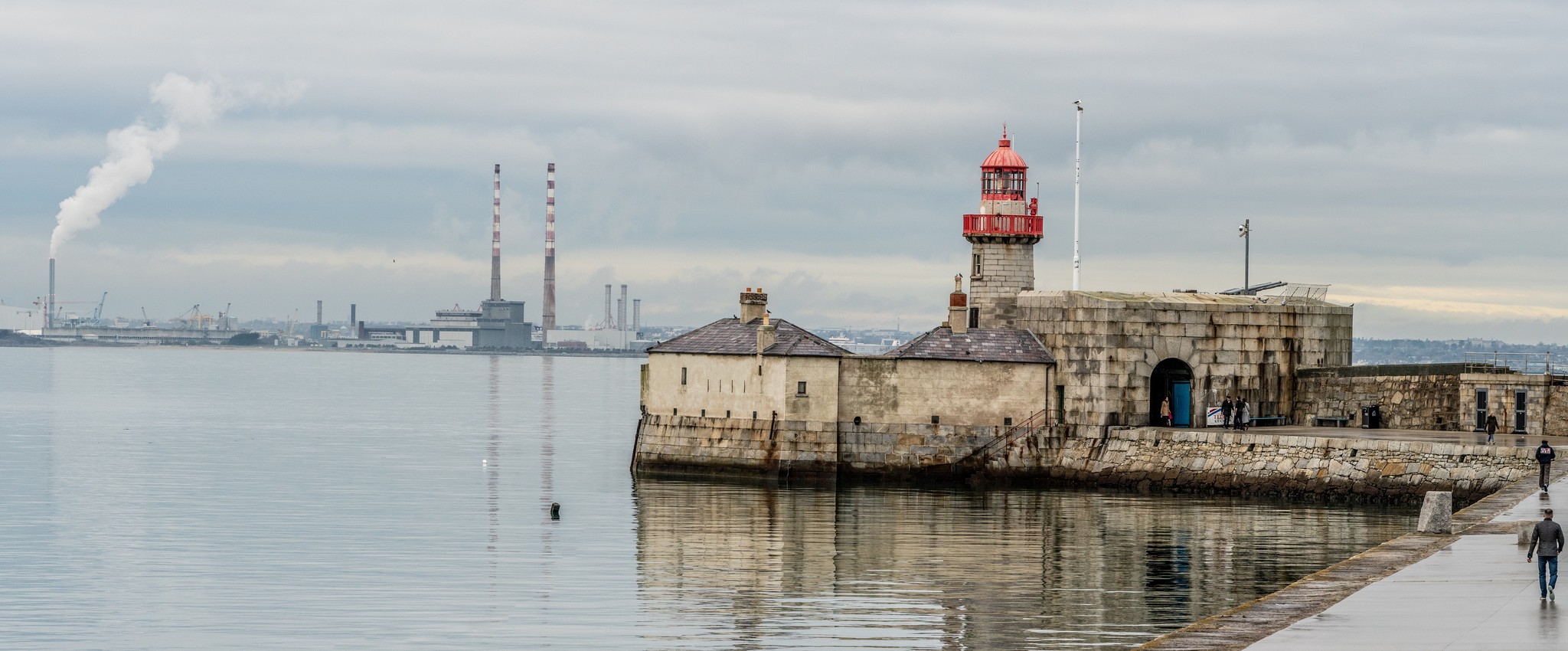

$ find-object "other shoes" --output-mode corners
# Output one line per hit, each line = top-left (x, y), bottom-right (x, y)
(1493, 441), (1495, 444)
(1541, 487), (1545, 491)
(1540, 596), (1546, 600)
(1544, 484), (1548, 492)
(1487, 441), (1491, 444)
(1548, 585), (1555, 601)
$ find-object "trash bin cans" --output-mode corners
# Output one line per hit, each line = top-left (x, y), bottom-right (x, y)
(1361, 405), (1379, 429)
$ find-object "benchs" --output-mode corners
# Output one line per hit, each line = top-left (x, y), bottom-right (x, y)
(1249, 418), (1286, 427)
(1310, 418), (1349, 428)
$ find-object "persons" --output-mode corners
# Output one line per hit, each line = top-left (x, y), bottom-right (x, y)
(1055, 419), (1060, 427)
(1231, 396), (1245, 431)
(1527, 509), (1564, 601)
(1535, 439), (1556, 493)
(1484, 411), (1499, 444)
(1242, 398), (1250, 430)
(1221, 396), (1235, 429)
(1160, 395), (1171, 427)
